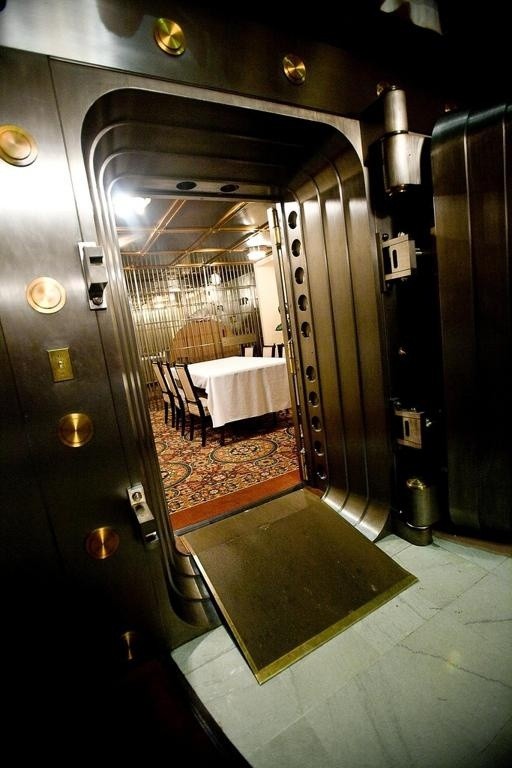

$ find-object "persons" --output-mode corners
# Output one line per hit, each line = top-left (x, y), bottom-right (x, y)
(216, 297), (248, 336)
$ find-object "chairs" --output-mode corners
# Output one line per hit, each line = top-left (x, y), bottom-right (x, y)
(243, 345), (257, 357)
(277, 343), (284, 357)
(173, 363), (210, 447)
(151, 360), (176, 428)
(262, 344), (276, 357)
(160, 360), (189, 436)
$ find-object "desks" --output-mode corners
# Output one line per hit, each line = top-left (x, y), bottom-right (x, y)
(170, 355), (299, 446)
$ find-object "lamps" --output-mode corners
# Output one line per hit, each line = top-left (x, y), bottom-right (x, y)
(247, 246), (267, 260)
